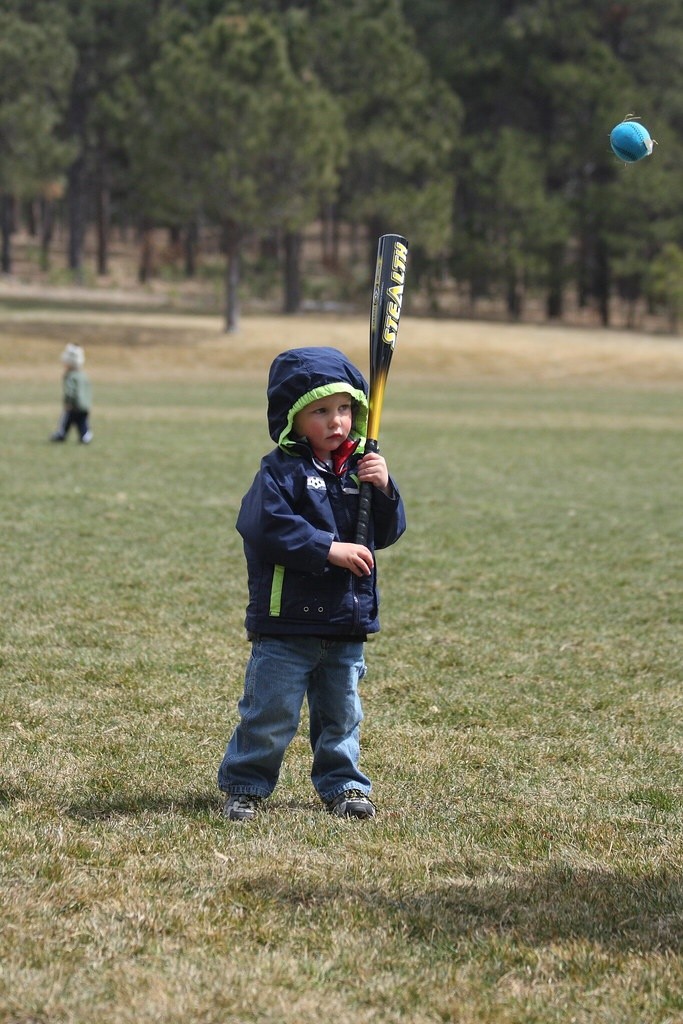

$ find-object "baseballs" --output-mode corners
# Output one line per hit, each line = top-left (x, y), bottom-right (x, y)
(609, 122), (656, 162)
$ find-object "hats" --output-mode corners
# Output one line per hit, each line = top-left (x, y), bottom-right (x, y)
(60, 342), (85, 366)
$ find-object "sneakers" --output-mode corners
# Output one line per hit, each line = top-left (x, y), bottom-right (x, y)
(328, 789), (377, 821)
(222, 791), (256, 822)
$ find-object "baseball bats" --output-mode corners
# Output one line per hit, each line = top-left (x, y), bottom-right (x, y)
(348, 231), (410, 544)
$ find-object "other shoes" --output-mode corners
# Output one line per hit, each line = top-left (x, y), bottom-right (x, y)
(81, 432), (91, 443)
(49, 435), (62, 442)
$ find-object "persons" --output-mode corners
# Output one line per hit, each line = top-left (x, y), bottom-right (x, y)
(50, 341), (92, 444)
(219, 347), (407, 819)
